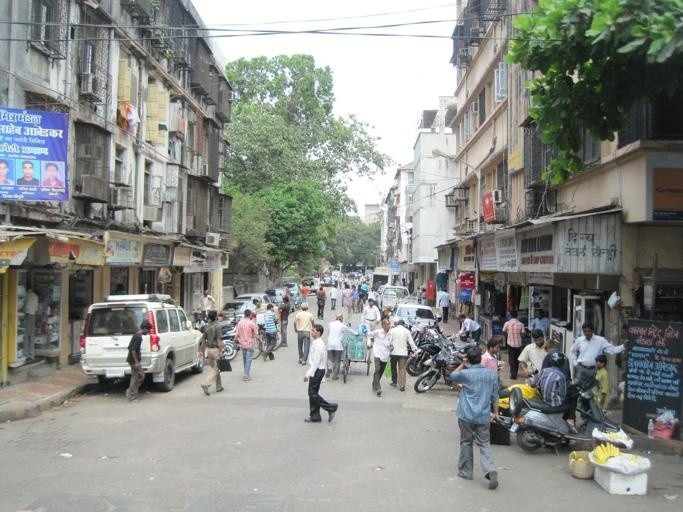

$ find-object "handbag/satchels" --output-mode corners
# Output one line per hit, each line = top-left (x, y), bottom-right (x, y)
(490, 422), (510, 445)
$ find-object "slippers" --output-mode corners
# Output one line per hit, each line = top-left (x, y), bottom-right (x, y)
(566, 421), (577, 433)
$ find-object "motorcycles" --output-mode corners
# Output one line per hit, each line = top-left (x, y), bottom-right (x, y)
(193, 313), (255, 362)
(406, 327), (481, 383)
(505, 379), (623, 456)
(413, 346), (511, 407)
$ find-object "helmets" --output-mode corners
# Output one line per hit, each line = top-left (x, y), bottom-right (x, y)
(549, 352), (564, 366)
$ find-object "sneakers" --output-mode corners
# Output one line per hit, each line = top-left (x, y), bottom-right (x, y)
(488, 471), (498, 489)
(217, 387), (224, 392)
(299, 346), (405, 397)
(458, 472), (472, 479)
(201, 384), (210, 396)
(328, 404), (337, 421)
(305, 417), (321, 422)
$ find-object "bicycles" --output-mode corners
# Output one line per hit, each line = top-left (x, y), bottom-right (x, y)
(248, 328), (285, 363)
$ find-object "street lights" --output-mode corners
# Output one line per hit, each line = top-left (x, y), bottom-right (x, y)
(428, 148), (482, 318)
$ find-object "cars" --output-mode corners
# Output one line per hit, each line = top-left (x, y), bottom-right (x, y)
(315, 276), (334, 288)
(385, 304), (439, 349)
(299, 278), (320, 296)
(235, 293), (271, 308)
(279, 282), (302, 306)
(265, 288), (295, 315)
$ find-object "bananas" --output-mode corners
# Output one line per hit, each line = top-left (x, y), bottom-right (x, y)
(592, 442), (621, 465)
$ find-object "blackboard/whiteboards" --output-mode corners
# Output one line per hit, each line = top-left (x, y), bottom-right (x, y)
(623, 318), (683, 441)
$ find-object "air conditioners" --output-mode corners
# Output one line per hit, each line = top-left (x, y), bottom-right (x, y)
(469, 102), (478, 115)
(228, 91), (235, 102)
(205, 232), (221, 248)
(110, 187), (133, 208)
(80, 72), (103, 102)
(79, 143), (102, 159)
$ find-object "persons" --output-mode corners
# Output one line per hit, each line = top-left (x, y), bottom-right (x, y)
(480, 337), (503, 374)
(501, 309), (630, 433)
(15, 160), (39, 185)
(245, 270), (482, 381)
(449, 344), (501, 491)
(231, 309), (261, 381)
(196, 310), (226, 396)
(42, 163), (63, 187)
(386, 319), (421, 391)
(124, 319), (152, 402)
(21, 284), (39, 360)
(197, 289), (216, 312)
(365, 317), (393, 396)
(0, 158), (14, 185)
(301, 324), (338, 422)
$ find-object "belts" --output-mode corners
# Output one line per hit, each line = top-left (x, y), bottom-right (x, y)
(579, 363), (595, 369)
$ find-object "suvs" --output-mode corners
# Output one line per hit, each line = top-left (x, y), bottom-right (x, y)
(378, 284), (410, 313)
(78, 292), (201, 392)
